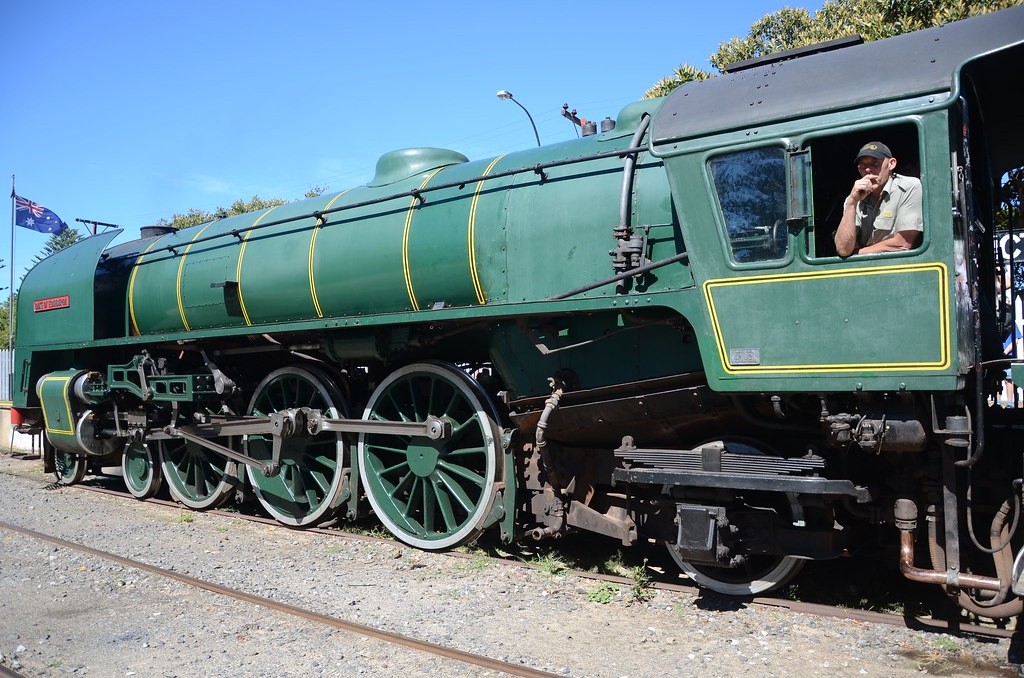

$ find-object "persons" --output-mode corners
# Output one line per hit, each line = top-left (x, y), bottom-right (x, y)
(834, 142), (923, 257)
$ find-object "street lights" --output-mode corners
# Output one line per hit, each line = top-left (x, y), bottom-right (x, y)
(497, 90), (542, 147)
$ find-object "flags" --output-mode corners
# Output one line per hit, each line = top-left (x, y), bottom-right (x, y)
(14, 194), (69, 236)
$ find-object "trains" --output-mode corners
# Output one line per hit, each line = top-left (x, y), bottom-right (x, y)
(8, 4), (1024, 664)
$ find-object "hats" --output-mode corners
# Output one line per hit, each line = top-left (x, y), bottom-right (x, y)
(854, 142), (892, 164)
